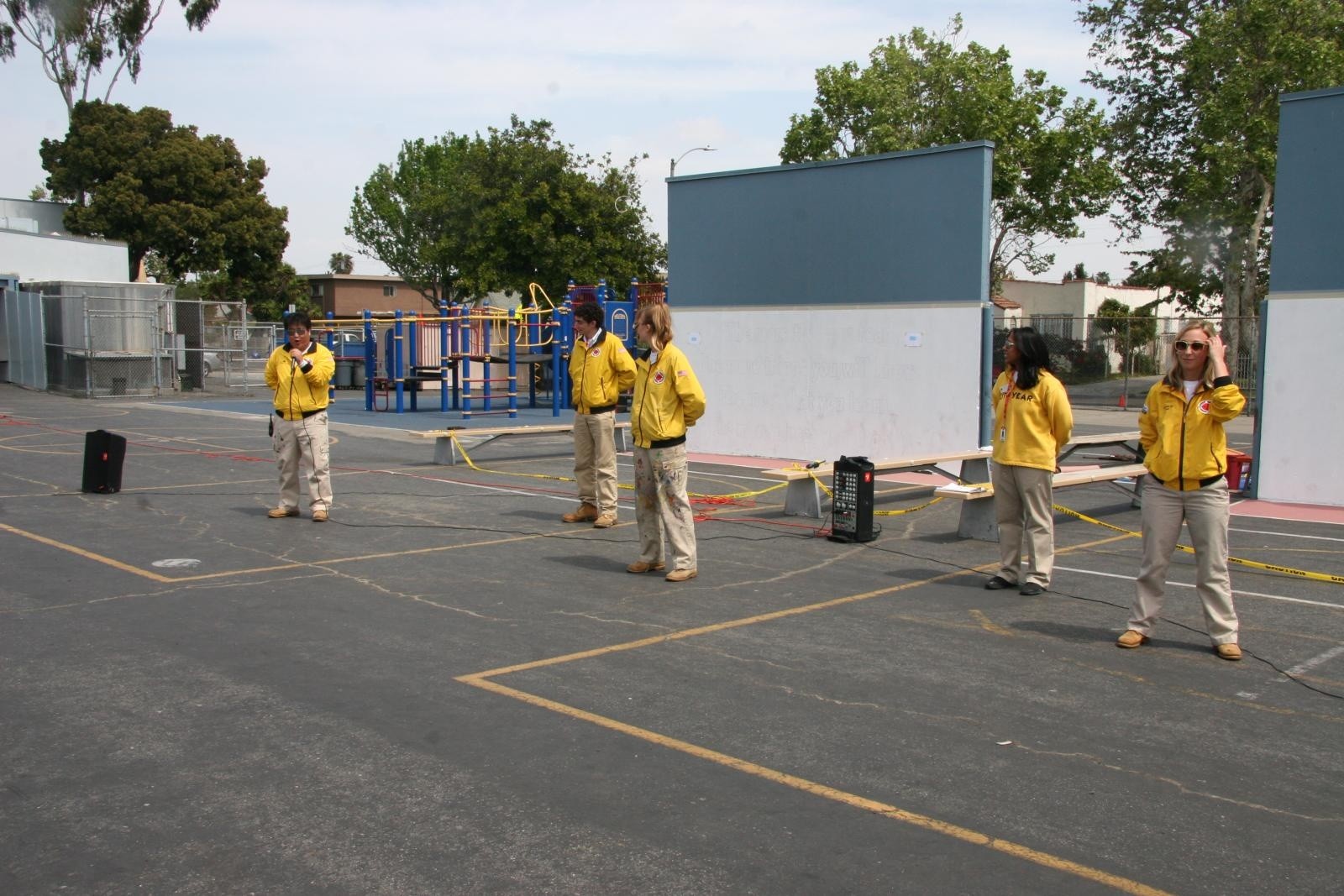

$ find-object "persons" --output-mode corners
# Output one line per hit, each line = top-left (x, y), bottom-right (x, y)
(985, 326), (1073, 595)
(265, 312), (336, 522)
(626, 303), (706, 581)
(562, 303), (637, 527)
(1116, 319), (1246, 660)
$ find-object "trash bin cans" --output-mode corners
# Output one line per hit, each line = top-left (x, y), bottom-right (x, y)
(1225, 455), (1253, 489)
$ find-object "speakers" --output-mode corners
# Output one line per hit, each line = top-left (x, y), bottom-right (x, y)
(82, 429), (126, 493)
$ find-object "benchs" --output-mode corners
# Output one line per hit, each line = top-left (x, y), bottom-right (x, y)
(411, 422), (634, 465)
(761, 432), (1150, 543)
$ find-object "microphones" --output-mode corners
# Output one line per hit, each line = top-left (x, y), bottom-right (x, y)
(291, 356), (297, 375)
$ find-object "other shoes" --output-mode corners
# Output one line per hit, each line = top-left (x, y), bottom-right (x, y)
(665, 568), (697, 581)
(269, 508), (294, 517)
(986, 576), (1010, 588)
(593, 514), (618, 528)
(1215, 643), (1241, 660)
(312, 510), (329, 521)
(1020, 582), (1043, 594)
(627, 560), (665, 573)
(562, 502), (598, 522)
(1118, 630), (1146, 647)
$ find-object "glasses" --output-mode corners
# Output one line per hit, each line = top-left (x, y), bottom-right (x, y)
(1174, 341), (1209, 352)
(1005, 340), (1015, 348)
(287, 329), (306, 335)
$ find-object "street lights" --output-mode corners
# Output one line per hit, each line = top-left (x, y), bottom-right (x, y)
(671, 147), (718, 178)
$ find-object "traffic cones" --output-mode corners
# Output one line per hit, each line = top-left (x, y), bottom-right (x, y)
(1118, 395), (1126, 407)
